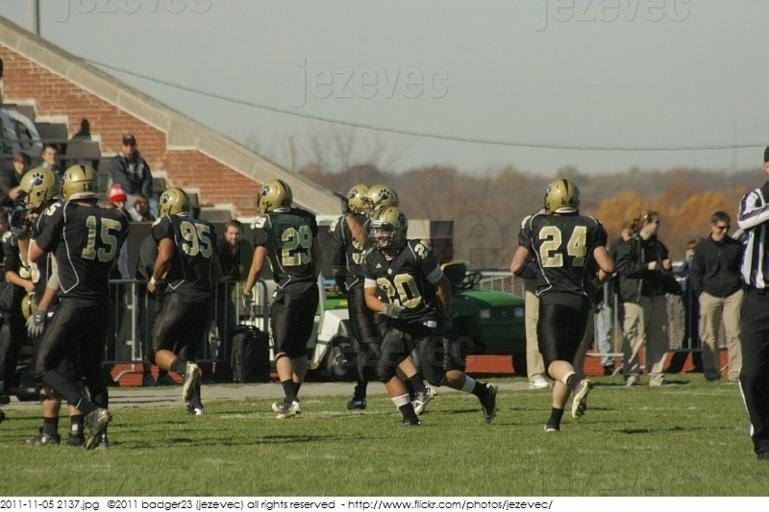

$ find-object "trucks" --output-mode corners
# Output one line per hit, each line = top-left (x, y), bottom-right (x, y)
(237, 210), (529, 379)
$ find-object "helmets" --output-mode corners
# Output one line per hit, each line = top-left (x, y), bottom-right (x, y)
(61, 164), (100, 203)
(543, 178), (582, 216)
(346, 184), (408, 250)
(21, 293), (41, 318)
(15, 168), (56, 210)
(159, 187), (190, 217)
(254, 179), (293, 215)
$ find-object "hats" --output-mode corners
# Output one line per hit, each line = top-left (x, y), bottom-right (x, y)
(122, 134), (136, 145)
(108, 184), (126, 204)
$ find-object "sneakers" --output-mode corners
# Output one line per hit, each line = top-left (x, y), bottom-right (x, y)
(571, 379), (593, 419)
(413, 385), (438, 416)
(271, 401), (291, 414)
(157, 373), (182, 386)
(185, 403), (205, 416)
(142, 375), (160, 387)
(478, 382), (500, 423)
(598, 363), (740, 389)
(276, 398), (302, 419)
(527, 377), (550, 390)
(346, 400), (366, 410)
(182, 360), (199, 403)
(25, 406), (113, 450)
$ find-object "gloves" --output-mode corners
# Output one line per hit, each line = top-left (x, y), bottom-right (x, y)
(378, 302), (401, 320)
(24, 309), (47, 340)
(240, 289), (255, 310)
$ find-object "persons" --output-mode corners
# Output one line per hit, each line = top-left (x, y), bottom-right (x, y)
(1, 62), (769, 462)
